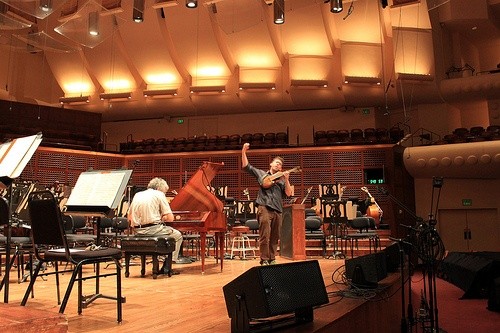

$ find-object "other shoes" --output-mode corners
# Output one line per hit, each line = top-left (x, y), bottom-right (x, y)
(261, 260), (269, 266)
(271, 260), (276, 264)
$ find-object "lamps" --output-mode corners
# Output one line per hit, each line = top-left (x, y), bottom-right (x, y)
(274, 0), (285, 24)
(39, 0), (52, 12)
(330, 0), (343, 13)
(132, 0), (144, 23)
(185, 0), (197, 9)
(88, 11), (100, 36)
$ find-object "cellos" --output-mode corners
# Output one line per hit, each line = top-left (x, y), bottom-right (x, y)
(360, 187), (384, 226)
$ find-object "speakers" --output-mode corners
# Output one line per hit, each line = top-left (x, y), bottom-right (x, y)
(222, 260), (329, 319)
(344, 241), (401, 288)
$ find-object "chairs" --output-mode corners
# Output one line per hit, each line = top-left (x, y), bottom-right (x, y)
(120, 131), (288, 155)
(313, 125), (500, 144)
(0, 190), (381, 324)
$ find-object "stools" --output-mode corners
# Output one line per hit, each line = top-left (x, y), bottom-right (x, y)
(230, 226), (256, 261)
(120, 236), (176, 280)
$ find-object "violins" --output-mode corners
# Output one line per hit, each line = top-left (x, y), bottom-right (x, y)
(257, 166), (303, 188)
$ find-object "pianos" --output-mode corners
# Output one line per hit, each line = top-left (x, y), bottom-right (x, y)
(160, 161), (228, 274)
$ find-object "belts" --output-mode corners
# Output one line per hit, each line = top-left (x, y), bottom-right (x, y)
(134, 223), (157, 228)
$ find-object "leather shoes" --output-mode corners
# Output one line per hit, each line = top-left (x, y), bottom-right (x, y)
(161, 268), (179, 274)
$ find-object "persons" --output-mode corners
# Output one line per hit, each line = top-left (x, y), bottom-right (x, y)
(242, 142), (291, 265)
(129, 178), (183, 262)
